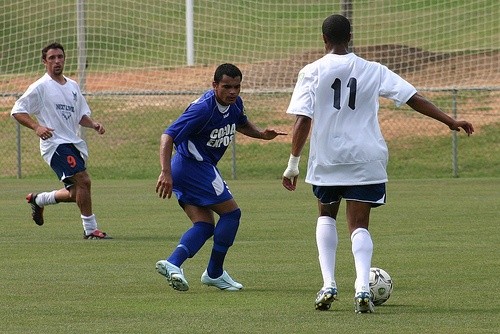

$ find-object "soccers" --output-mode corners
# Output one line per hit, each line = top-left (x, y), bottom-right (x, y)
(354, 267), (393, 306)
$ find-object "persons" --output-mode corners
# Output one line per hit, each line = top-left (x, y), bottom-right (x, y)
(10, 43), (107, 239)
(281, 14), (474, 314)
(153, 63), (288, 291)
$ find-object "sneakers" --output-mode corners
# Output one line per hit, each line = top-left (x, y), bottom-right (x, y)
(155, 260), (189, 291)
(84, 230), (113, 239)
(355, 285), (375, 313)
(26, 192), (44, 226)
(314, 280), (337, 311)
(201, 268), (243, 291)
